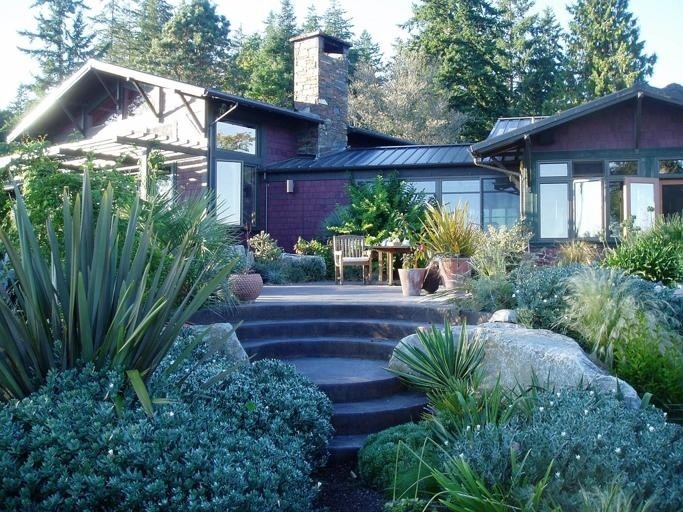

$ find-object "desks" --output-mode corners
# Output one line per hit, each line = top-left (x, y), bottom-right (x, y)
(365, 246), (411, 286)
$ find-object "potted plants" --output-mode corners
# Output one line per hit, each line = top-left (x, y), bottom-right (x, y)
(224, 222), (263, 302)
(397, 194), (480, 296)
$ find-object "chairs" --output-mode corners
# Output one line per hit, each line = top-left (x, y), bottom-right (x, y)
(331, 235), (372, 285)
